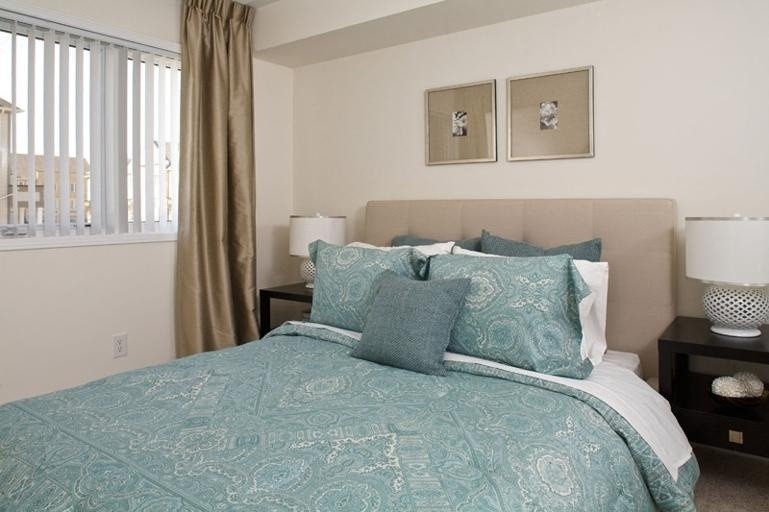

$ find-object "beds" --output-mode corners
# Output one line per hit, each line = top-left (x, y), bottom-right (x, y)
(1, 199), (680, 511)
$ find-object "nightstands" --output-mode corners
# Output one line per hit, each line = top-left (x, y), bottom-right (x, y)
(259, 283), (314, 339)
(658, 315), (769, 464)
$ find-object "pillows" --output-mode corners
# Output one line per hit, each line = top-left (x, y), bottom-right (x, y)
(419, 254), (596, 380)
(346, 241), (457, 259)
(307, 240), (427, 333)
(349, 269), (471, 377)
(390, 235), (481, 252)
(479, 226), (602, 263)
(453, 246), (609, 366)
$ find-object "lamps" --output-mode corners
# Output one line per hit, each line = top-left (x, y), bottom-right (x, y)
(288, 215), (347, 288)
(685, 217), (769, 338)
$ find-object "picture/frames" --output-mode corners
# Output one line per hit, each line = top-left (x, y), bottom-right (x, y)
(424, 79), (498, 166)
(506, 65), (595, 162)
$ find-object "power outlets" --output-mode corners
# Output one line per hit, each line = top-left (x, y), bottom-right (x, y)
(112, 334), (128, 358)
